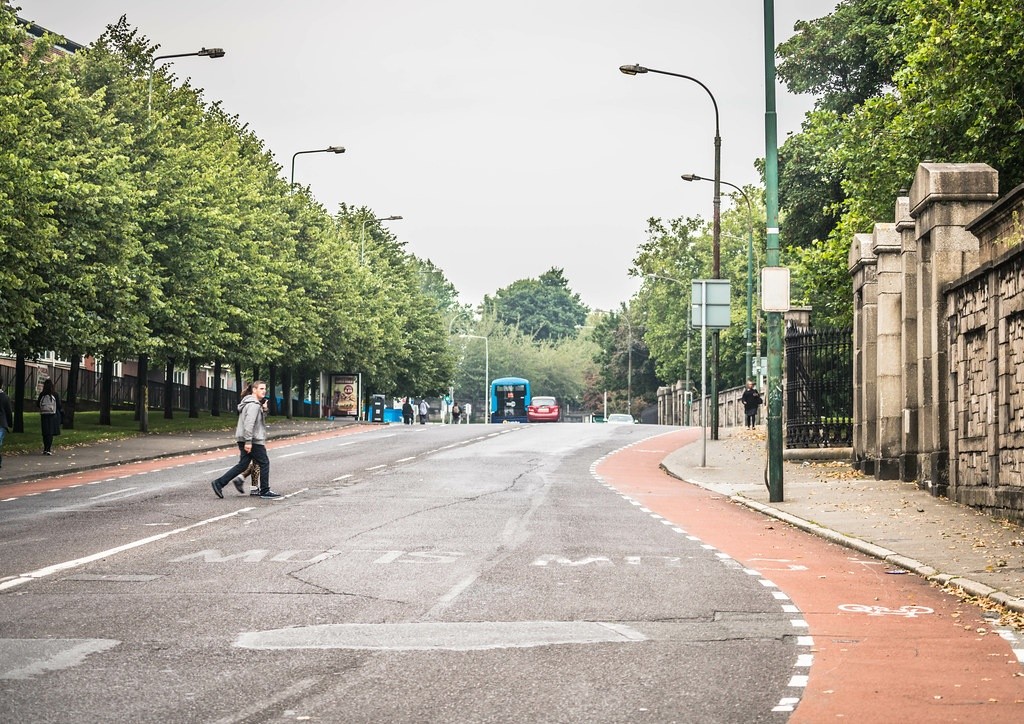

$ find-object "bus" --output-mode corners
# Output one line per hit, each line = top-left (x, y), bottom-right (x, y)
(489, 376), (535, 423)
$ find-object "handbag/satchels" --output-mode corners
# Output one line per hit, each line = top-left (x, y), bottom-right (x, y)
(757, 397), (763, 404)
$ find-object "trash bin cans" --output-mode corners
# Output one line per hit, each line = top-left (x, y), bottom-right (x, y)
(372, 397), (384, 422)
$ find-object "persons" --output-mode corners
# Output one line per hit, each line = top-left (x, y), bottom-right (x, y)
(212, 381), (281, 498)
(420, 399), (430, 424)
(37, 380), (61, 455)
(452, 403), (462, 424)
(233, 385), (268, 497)
(402, 399), (412, 424)
(742, 381), (760, 430)
(0, 375), (13, 467)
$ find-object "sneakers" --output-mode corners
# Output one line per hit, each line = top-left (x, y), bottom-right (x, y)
(212, 480), (224, 498)
(260, 490), (280, 498)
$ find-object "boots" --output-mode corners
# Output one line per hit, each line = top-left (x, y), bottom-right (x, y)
(233, 475), (246, 493)
(250, 486), (261, 496)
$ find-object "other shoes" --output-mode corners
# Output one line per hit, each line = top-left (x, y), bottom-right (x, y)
(747, 427), (750, 430)
(752, 427), (756, 430)
(43, 451), (47, 454)
(47, 451), (52, 456)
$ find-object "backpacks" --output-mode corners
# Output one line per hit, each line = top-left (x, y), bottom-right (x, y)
(39, 394), (57, 415)
(454, 406), (460, 414)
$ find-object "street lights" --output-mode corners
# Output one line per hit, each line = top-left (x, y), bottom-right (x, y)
(679, 172), (753, 384)
(706, 229), (761, 426)
(647, 273), (692, 427)
(593, 308), (632, 414)
(284, 145), (347, 420)
(355, 215), (404, 422)
(447, 312), (469, 424)
(617, 62), (723, 442)
(573, 324), (608, 424)
(135, 45), (228, 435)
(457, 334), (489, 424)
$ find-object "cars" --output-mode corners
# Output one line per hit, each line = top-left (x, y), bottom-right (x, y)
(602, 413), (639, 425)
(527, 396), (561, 423)
